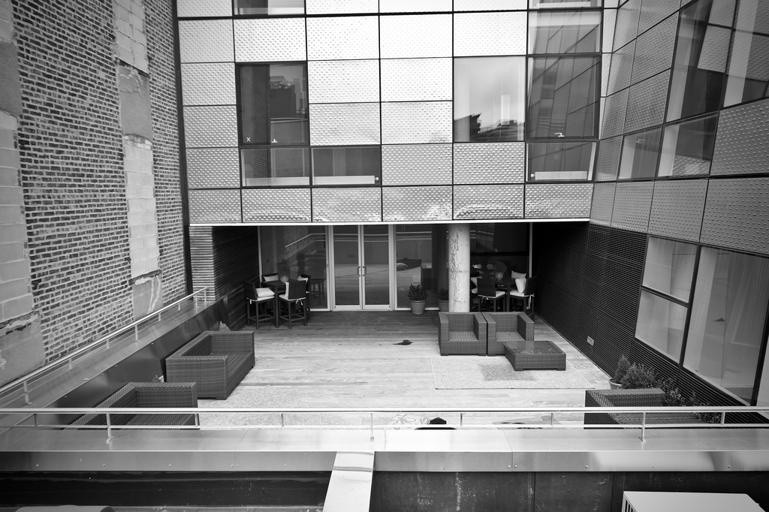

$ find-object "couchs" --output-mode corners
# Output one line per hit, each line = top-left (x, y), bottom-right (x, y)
(57, 381), (204, 434)
(437, 311), (487, 358)
(483, 311), (537, 358)
(396, 256), (422, 271)
(582, 386), (705, 431)
(166, 330), (255, 401)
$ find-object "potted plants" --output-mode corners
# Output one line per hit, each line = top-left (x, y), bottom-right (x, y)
(406, 283), (429, 317)
(608, 354), (630, 390)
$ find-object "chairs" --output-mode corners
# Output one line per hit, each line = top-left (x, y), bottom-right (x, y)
(243, 273), (310, 329)
(288, 245), (326, 308)
(469, 264), (538, 322)
